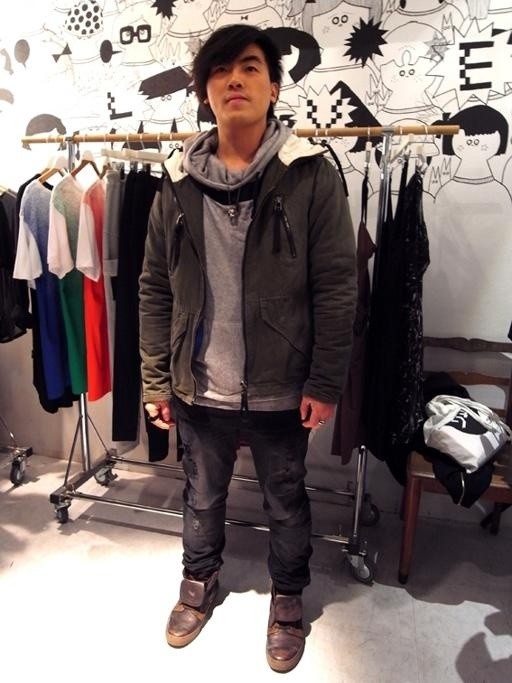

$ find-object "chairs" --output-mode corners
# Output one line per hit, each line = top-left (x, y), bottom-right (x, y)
(394, 337), (512, 586)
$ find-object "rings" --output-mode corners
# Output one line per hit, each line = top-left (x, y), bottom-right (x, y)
(316, 417), (326, 426)
(147, 413), (161, 423)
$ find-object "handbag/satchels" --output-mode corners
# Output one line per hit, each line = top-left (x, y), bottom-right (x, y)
(423, 394), (511, 475)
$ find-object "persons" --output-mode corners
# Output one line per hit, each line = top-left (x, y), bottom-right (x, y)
(136, 23), (358, 673)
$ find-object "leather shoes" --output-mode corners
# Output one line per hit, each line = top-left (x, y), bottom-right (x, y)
(166, 571), (220, 647)
(266, 583), (305, 673)
